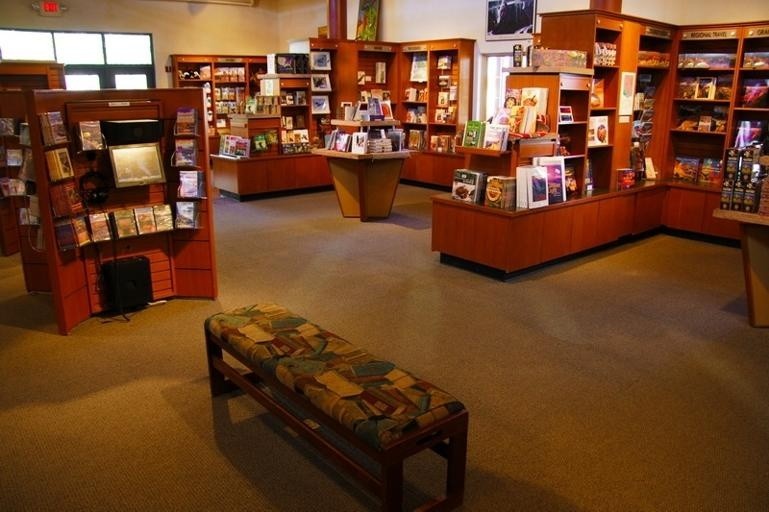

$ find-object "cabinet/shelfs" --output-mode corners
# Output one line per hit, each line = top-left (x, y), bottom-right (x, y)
(311, 118), (419, 222)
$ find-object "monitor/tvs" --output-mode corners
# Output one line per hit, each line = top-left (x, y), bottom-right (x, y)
(108, 142), (167, 189)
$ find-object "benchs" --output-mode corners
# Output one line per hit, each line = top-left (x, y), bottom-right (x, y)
(204, 300), (469, 511)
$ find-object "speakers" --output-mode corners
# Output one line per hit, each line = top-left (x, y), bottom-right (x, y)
(100, 256), (153, 312)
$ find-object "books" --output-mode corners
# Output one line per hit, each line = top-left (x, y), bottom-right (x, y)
(1, 104), (202, 253)
(172, 43), (458, 159)
(450, 43), (769, 222)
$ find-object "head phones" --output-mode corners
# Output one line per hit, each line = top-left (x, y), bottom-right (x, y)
(79, 171), (111, 205)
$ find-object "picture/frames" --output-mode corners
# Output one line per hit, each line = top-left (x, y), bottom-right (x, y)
(355, 0), (380, 42)
